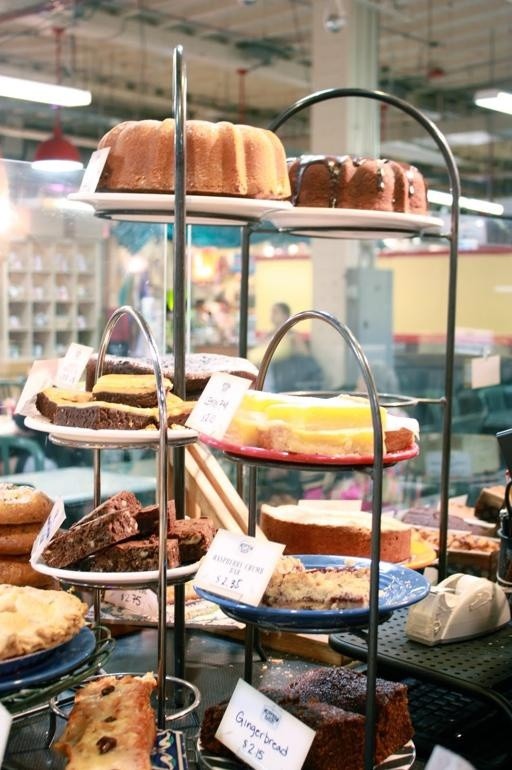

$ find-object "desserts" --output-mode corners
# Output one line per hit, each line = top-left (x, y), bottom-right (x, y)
(1, 478), (506, 769)
(36, 349), (417, 457)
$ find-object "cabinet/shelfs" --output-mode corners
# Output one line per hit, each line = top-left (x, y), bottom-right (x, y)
(2, 227), (104, 379)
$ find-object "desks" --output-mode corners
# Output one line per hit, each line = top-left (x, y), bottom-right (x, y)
(1, 610), (361, 769)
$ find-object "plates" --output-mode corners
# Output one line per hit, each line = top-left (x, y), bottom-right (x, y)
(262, 204), (446, 243)
(0, 623), (96, 695)
(68, 188), (294, 228)
(22, 417), (200, 444)
(194, 431), (419, 467)
(29, 530), (204, 586)
(0, 530), (436, 712)
(191, 730), (417, 770)
(193, 553), (433, 633)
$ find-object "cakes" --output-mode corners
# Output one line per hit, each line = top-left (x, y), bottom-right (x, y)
(94, 118), (291, 198)
(286, 151), (428, 213)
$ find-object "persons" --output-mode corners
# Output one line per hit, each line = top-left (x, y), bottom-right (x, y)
(247, 301), (307, 365)
(189, 292), (257, 349)
